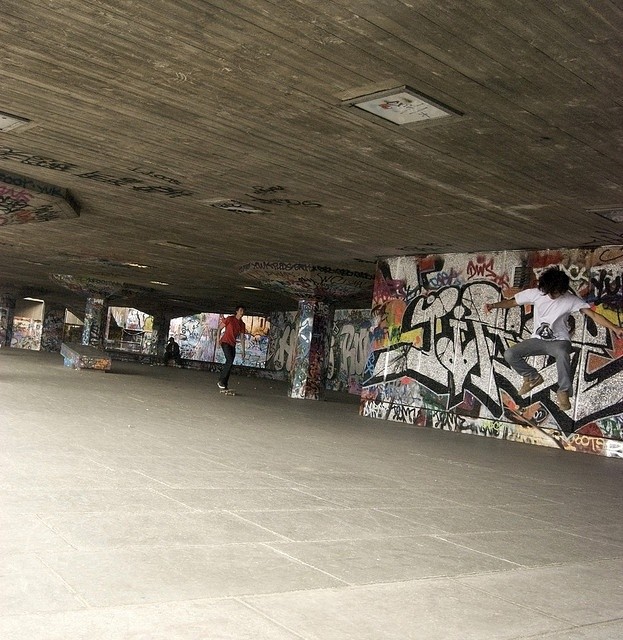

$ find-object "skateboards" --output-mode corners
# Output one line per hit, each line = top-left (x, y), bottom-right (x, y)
(220, 389), (236, 396)
(505, 408), (564, 449)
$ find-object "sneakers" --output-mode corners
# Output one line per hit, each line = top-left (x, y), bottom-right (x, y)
(556, 388), (572, 411)
(216, 381), (226, 389)
(517, 373), (546, 395)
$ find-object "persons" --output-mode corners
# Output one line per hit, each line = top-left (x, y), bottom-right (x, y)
(483, 264), (623, 412)
(161, 337), (183, 368)
(216, 305), (246, 393)
